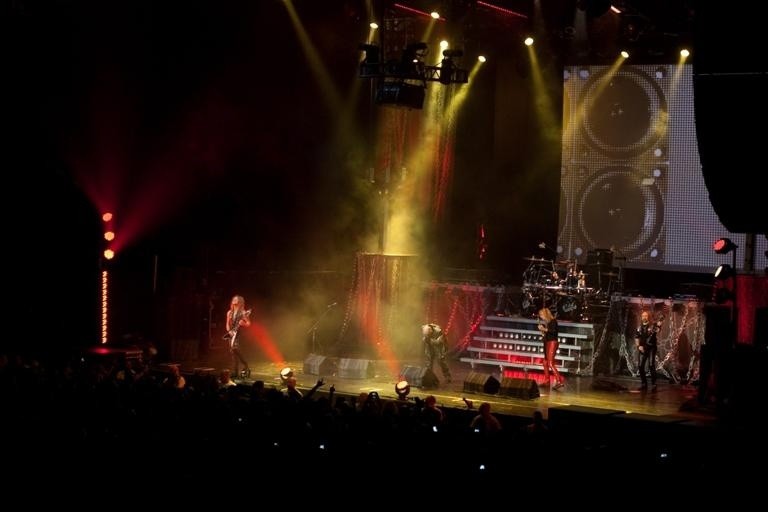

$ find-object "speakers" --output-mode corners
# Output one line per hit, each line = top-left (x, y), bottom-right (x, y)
(556, 64), (768, 274)
(463, 372), (500, 395)
(399, 364), (439, 390)
(303, 355), (332, 376)
(338, 358), (375, 379)
(498, 377), (540, 400)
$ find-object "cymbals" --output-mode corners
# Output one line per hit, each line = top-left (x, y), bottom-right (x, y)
(524, 257), (552, 264)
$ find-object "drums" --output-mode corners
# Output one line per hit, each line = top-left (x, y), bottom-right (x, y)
(521, 290), (552, 317)
(556, 295), (581, 321)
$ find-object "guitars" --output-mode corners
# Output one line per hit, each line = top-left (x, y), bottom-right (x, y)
(224, 310), (253, 347)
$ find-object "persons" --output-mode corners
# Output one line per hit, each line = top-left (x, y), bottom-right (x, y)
(225, 295), (250, 379)
(638, 311), (657, 394)
(537, 307), (567, 390)
(2, 359), (547, 438)
(421, 323), (452, 382)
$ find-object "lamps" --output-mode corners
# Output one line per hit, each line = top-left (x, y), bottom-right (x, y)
(712, 233), (739, 352)
(393, 381), (411, 398)
(280, 365), (293, 385)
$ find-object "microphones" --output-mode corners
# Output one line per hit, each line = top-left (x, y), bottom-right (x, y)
(327, 302), (337, 308)
